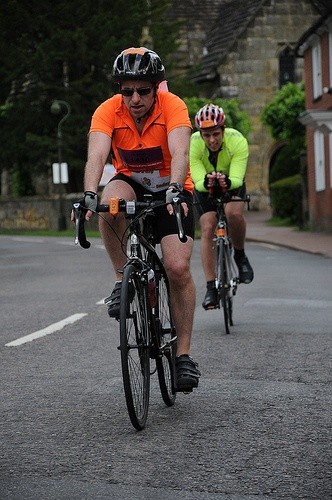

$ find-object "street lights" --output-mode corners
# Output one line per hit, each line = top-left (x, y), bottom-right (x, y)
(49, 99), (71, 230)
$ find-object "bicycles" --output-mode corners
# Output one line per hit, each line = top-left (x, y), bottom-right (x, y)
(193, 190), (250, 335)
(71, 194), (198, 430)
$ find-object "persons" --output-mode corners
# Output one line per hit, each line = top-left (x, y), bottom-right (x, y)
(190, 103), (254, 307)
(70, 48), (200, 386)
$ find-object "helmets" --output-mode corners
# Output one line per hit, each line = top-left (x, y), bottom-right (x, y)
(112, 46), (165, 83)
(194, 103), (225, 129)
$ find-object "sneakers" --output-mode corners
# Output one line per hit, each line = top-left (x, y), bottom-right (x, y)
(174, 354), (200, 389)
(104, 282), (136, 318)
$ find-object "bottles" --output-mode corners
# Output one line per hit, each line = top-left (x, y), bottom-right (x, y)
(148, 269), (156, 307)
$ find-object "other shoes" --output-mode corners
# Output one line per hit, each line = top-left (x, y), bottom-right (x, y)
(233, 255), (253, 284)
(202, 287), (218, 308)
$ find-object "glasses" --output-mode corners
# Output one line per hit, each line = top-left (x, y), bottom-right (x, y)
(119, 85), (156, 96)
